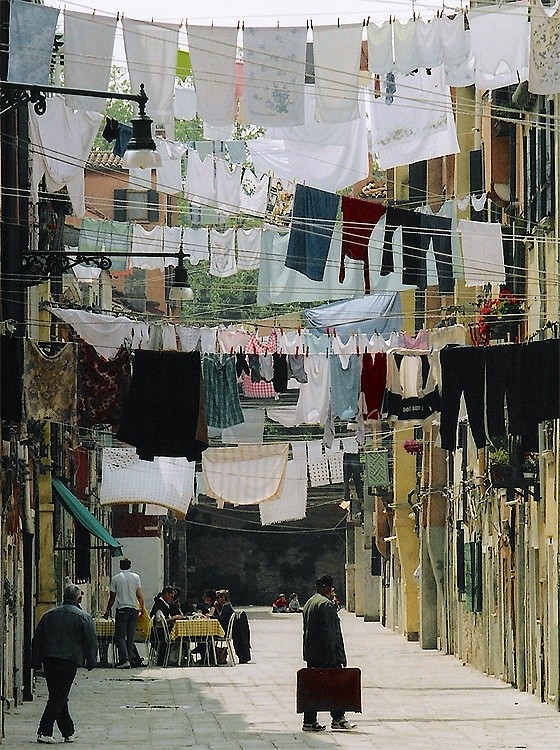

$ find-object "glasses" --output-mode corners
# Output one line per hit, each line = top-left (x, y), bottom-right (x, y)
(167, 594), (174, 599)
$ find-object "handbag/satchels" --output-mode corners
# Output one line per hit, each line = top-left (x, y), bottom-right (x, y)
(209, 639), (228, 664)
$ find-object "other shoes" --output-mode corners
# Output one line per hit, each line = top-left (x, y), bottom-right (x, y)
(37, 736), (58, 745)
(191, 645), (204, 654)
(65, 730), (79, 742)
(117, 662), (130, 669)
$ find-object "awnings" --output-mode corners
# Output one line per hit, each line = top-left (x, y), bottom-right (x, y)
(51, 476), (124, 550)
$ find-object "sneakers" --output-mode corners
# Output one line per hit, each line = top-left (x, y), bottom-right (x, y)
(331, 719), (357, 730)
(302, 722), (326, 732)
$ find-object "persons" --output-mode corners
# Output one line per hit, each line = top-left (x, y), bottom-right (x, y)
(302, 575), (358, 731)
(104, 558), (145, 669)
(289, 593), (303, 613)
(149, 584), (235, 666)
(273, 594), (289, 613)
(30, 584), (98, 744)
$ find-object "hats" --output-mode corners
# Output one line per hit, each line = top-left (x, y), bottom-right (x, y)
(279, 594), (284, 597)
(316, 575), (333, 586)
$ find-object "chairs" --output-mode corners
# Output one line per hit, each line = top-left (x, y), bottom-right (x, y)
(91, 611), (118, 668)
(132, 603), (194, 667)
(196, 605), (239, 666)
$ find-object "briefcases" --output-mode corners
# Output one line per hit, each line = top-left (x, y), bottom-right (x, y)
(296, 668), (362, 714)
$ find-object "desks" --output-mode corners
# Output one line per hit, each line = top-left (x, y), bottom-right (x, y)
(91, 617), (118, 668)
(172, 619), (225, 666)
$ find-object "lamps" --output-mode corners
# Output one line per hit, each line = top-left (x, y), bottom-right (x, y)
(0, 82), (162, 171)
(23, 247), (193, 301)
